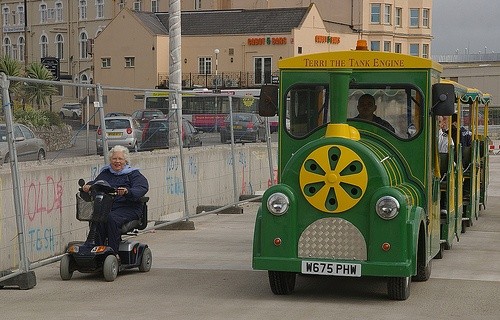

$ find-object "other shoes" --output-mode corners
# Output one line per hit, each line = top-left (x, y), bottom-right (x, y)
(440, 208), (447, 216)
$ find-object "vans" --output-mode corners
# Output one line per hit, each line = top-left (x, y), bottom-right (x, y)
(95, 112), (142, 155)
(60, 102), (82, 121)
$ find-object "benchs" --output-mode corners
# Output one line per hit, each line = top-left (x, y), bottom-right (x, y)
(440, 135), (485, 191)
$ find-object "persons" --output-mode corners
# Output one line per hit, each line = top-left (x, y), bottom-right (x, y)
(437, 111), (473, 154)
(353, 93), (396, 133)
(79, 144), (149, 257)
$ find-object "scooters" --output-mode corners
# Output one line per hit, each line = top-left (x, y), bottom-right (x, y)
(58, 176), (153, 281)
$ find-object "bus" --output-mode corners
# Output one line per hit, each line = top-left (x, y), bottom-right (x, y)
(250, 28), (493, 302)
(143, 89), (280, 135)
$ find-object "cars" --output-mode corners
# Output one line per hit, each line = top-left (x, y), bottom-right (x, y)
(0, 124), (46, 166)
(219, 111), (267, 144)
(104, 109), (165, 132)
(138, 115), (203, 151)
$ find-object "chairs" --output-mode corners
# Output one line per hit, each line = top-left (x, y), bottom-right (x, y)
(120, 197), (150, 234)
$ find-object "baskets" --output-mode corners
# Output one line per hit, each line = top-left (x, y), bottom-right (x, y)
(75, 191), (113, 223)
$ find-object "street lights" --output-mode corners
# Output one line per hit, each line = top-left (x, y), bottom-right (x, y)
(214, 48), (220, 134)
